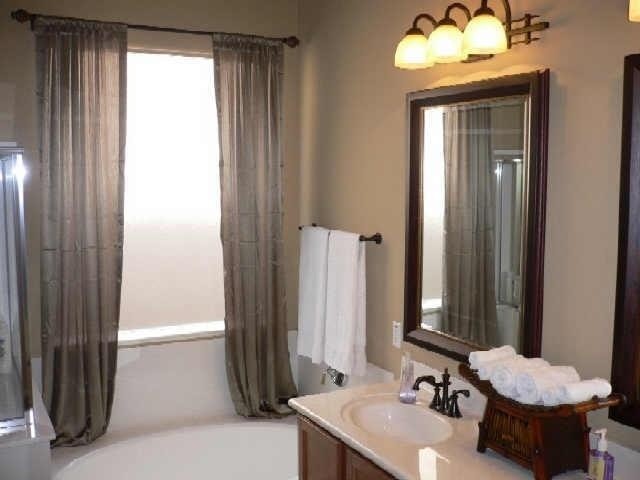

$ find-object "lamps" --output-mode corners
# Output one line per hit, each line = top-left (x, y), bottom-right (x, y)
(393, 0), (553, 74)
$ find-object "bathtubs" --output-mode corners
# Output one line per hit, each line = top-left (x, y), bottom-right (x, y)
(49, 405), (299, 480)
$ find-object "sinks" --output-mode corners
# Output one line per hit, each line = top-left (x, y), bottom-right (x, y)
(340, 392), (454, 446)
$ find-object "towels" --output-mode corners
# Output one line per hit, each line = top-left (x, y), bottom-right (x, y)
(321, 228), (373, 379)
(465, 339), (616, 415)
(293, 222), (330, 367)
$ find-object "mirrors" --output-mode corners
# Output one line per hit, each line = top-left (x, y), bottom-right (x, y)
(401, 68), (550, 365)
(607, 53), (640, 428)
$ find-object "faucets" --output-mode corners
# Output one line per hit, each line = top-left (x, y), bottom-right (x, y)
(411, 365), (452, 410)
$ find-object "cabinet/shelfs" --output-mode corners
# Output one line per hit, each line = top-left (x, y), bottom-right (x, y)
(340, 435), (394, 479)
(291, 412), (343, 480)
